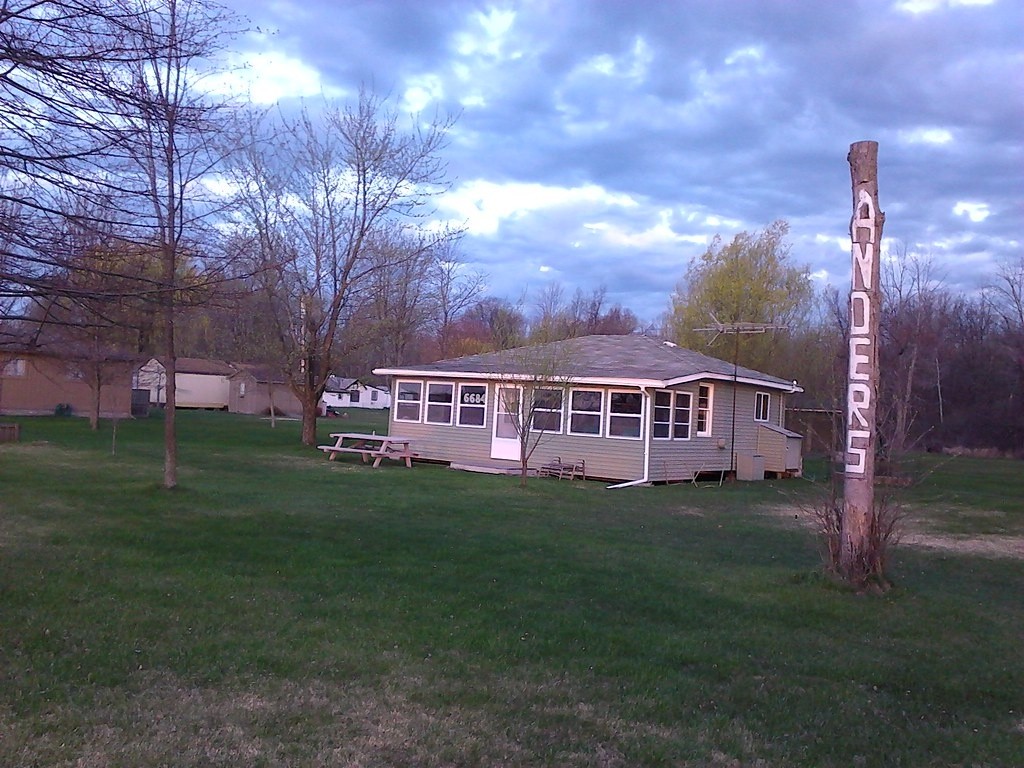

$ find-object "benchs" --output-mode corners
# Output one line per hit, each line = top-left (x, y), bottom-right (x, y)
(365, 445), (421, 458)
(536, 457), (587, 480)
(317, 445), (390, 457)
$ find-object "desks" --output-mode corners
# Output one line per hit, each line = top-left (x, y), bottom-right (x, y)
(328, 433), (416, 469)
